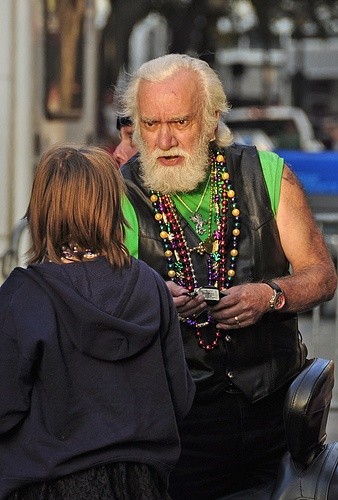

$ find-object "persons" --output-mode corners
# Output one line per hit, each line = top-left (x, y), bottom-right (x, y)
(118, 54), (338, 500)
(1, 143), (196, 500)
(316, 112), (338, 151)
(111, 113), (139, 168)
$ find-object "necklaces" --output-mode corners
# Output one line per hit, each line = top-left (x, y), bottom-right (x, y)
(140, 143), (236, 351)
(51, 246), (101, 260)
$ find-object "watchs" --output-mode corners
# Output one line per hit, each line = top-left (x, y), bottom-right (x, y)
(264, 281), (286, 319)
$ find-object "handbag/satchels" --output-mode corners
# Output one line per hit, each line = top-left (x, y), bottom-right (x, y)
(285, 358), (334, 471)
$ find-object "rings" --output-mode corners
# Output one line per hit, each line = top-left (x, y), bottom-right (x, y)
(235, 317), (240, 325)
